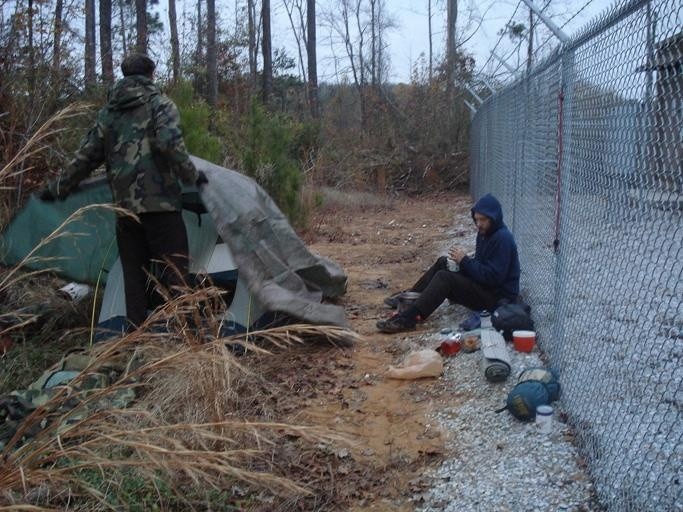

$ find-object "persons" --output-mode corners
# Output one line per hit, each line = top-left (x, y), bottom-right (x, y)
(27, 50), (212, 334)
(370, 191), (522, 335)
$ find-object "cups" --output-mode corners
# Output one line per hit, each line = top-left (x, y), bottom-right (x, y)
(535, 405), (554, 433)
(447, 258), (460, 272)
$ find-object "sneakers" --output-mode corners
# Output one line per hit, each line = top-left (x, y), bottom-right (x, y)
(376, 289), (416, 333)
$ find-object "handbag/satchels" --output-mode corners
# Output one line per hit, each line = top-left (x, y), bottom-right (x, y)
(491, 303), (534, 343)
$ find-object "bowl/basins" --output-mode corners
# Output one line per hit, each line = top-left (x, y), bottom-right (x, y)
(512, 331), (536, 353)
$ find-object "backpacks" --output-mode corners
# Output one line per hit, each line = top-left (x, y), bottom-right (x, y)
(495, 366), (561, 422)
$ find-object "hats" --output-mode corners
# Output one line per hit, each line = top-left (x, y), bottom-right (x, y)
(120, 52), (155, 76)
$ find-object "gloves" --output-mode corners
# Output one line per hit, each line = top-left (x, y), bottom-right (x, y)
(197, 170), (208, 187)
(39, 189), (56, 204)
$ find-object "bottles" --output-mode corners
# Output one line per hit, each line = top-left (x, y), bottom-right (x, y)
(480, 310), (492, 329)
(441, 332), (463, 355)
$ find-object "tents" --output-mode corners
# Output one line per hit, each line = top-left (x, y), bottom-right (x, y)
(1, 153), (347, 357)
